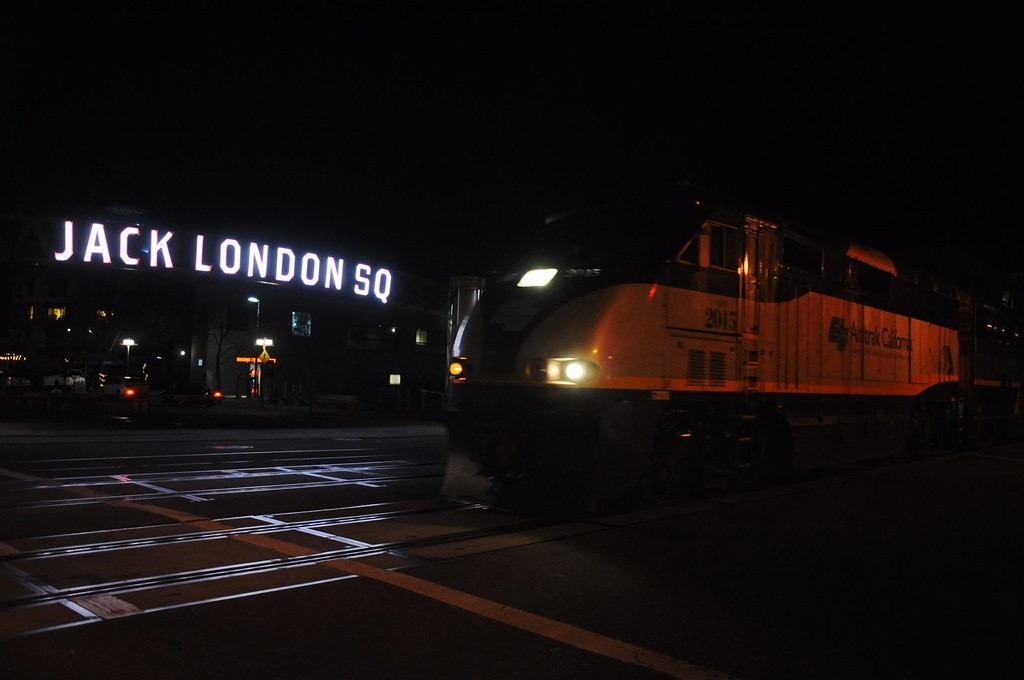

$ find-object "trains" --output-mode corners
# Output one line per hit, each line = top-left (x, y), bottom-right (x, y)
(436, 200), (1024, 518)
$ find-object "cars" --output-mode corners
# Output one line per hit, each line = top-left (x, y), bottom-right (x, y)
(40, 367), (86, 392)
(104, 375), (141, 400)
(159, 381), (225, 407)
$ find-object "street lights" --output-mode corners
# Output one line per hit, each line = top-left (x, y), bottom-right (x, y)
(119, 337), (138, 380)
(248, 296), (260, 355)
